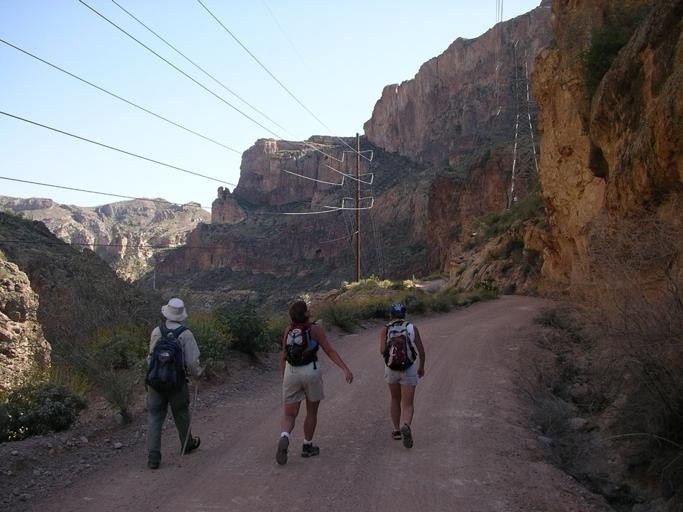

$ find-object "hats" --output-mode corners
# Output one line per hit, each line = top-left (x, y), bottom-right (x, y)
(389, 304), (406, 317)
(162, 298), (188, 320)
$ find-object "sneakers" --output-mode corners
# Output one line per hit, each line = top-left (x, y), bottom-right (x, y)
(392, 429), (403, 439)
(402, 425), (413, 448)
(275, 435), (289, 464)
(301, 442), (320, 457)
(147, 450), (162, 469)
(180, 436), (201, 454)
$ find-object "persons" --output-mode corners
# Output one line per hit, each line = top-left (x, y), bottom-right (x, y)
(378, 303), (426, 448)
(144, 298), (200, 469)
(276, 300), (353, 466)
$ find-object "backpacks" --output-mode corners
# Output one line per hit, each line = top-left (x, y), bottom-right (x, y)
(143, 321), (189, 392)
(382, 320), (417, 371)
(284, 322), (319, 370)
